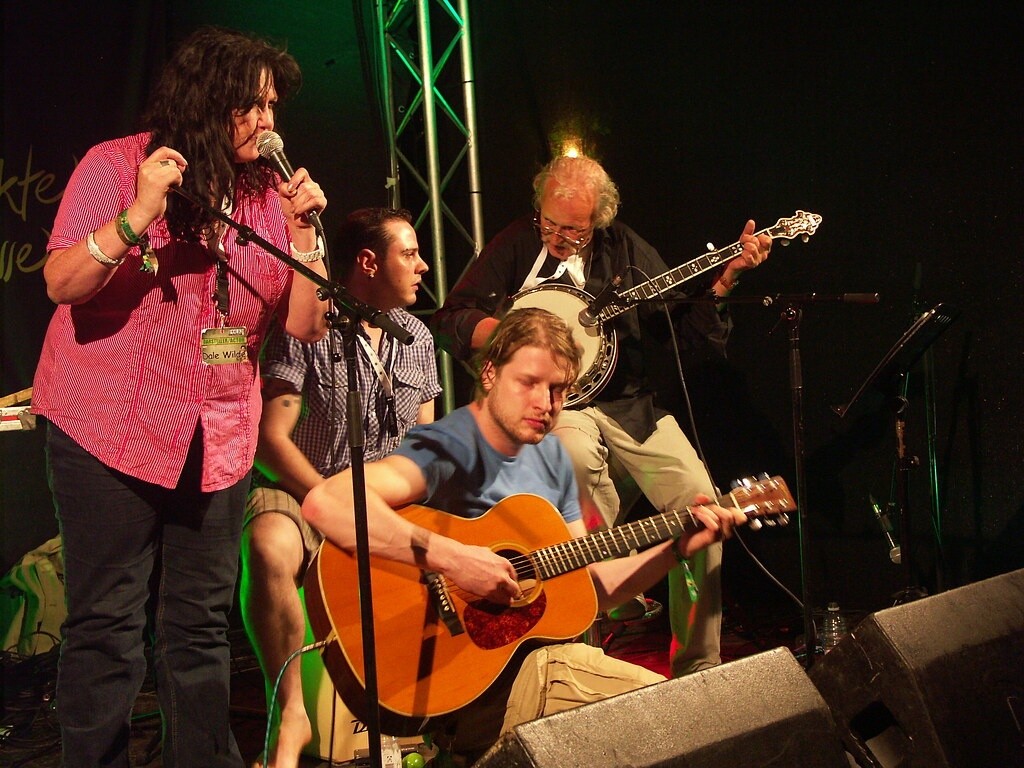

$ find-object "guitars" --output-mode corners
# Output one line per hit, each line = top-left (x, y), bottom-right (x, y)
(303, 472), (797, 736)
(498, 205), (824, 410)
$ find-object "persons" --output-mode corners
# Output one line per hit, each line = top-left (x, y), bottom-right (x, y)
(434, 134), (775, 683)
(240, 204), (442, 767)
(302, 299), (749, 768)
(22, 22), (335, 768)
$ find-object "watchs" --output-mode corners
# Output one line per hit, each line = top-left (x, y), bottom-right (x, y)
(288, 235), (327, 263)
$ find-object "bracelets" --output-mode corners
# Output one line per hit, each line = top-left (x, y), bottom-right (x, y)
(86, 230), (119, 266)
(717, 272), (740, 291)
(121, 208), (159, 275)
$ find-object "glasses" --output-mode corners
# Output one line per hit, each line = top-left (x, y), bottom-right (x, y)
(532, 211), (594, 248)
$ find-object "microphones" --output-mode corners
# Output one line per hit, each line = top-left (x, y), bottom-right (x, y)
(578, 266), (634, 328)
(867, 494), (906, 564)
(256, 132), (325, 232)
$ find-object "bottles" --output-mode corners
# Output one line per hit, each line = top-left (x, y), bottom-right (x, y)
(823, 602), (848, 655)
(379, 733), (402, 768)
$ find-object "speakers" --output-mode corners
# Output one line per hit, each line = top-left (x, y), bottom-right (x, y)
(809, 568), (1024, 768)
(470, 646), (850, 767)
